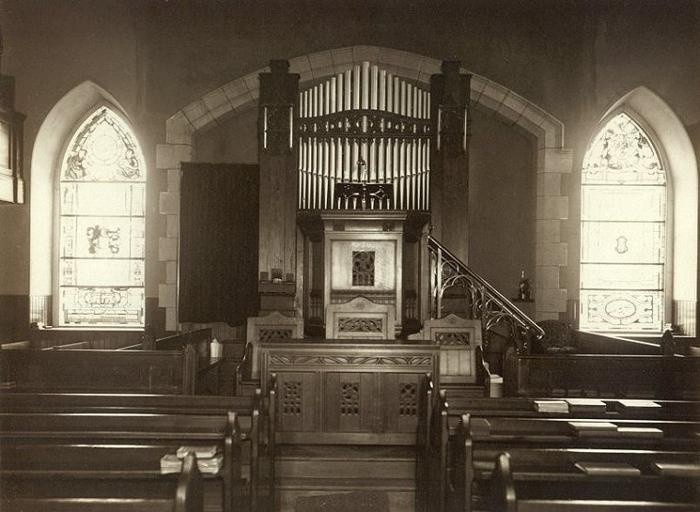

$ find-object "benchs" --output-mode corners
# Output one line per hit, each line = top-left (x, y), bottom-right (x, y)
(412, 373), (699, 510)
(0, 373), (278, 512)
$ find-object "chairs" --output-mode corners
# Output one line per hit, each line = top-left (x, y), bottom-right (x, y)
(234, 296), (505, 398)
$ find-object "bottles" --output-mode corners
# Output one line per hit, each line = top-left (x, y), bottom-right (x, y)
(520, 270), (529, 299)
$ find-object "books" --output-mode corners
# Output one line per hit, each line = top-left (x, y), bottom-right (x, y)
(534, 399), (570, 414)
(159, 445), (224, 474)
(568, 421), (618, 430)
(565, 399), (607, 414)
(618, 427), (664, 440)
(573, 430), (619, 439)
(647, 461), (699, 477)
(615, 399), (663, 416)
(574, 461), (641, 477)
